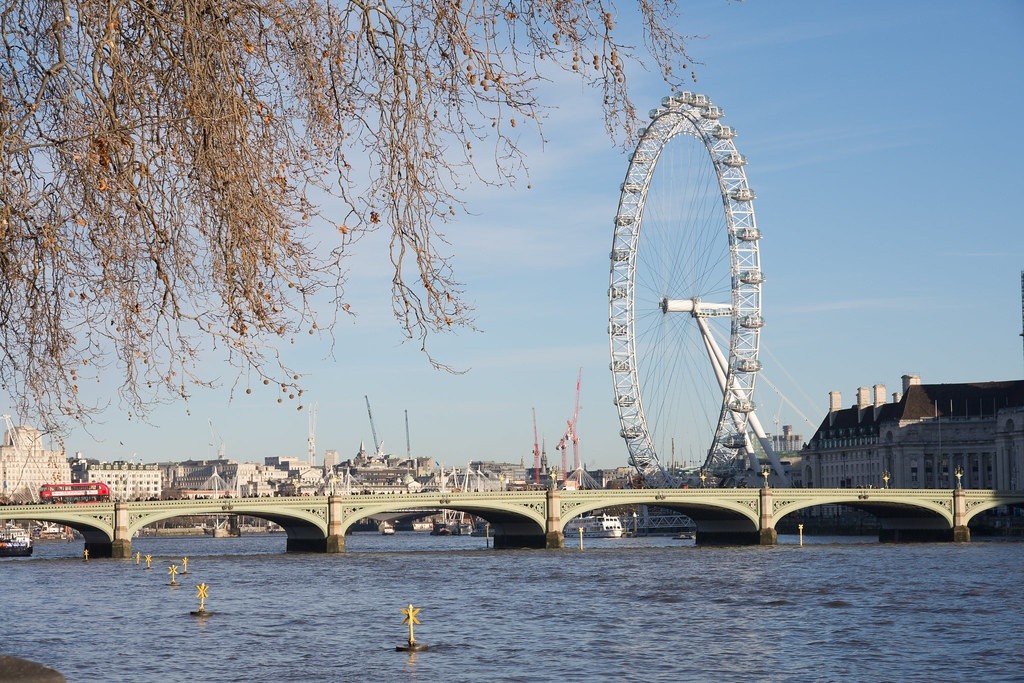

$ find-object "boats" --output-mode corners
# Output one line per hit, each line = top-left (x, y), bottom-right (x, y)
(383, 525), (395, 535)
(563, 513), (622, 538)
(430, 521), (453, 536)
(0, 520), (33, 557)
(672, 534), (696, 539)
(33, 521), (84, 542)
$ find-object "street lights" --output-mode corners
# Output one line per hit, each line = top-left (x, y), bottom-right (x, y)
(329, 472), (340, 496)
(881, 467), (890, 489)
(547, 464), (560, 490)
(762, 463), (771, 488)
(699, 468), (708, 487)
(294, 481), (301, 497)
(955, 464), (964, 487)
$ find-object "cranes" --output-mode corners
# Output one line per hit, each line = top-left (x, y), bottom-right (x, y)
(365, 395), (384, 456)
(311, 400), (318, 467)
(207, 418), (224, 460)
(404, 409), (411, 459)
(307, 402), (312, 466)
(532, 407), (541, 485)
(567, 367), (583, 483)
(556, 405), (583, 486)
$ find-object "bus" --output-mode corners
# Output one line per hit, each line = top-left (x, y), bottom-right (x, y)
(39, 481), (111, 507)
